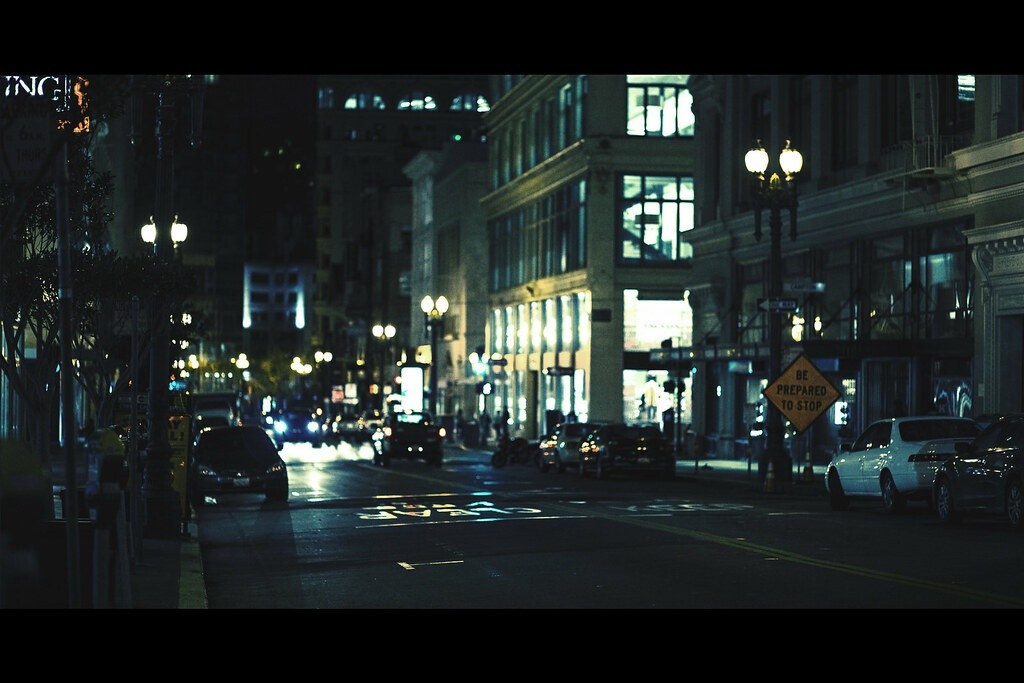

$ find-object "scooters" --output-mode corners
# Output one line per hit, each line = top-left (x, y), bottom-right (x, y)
(491, 432), (528, 468)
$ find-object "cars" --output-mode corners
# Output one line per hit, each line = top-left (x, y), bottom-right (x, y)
(825, 415), (986, 516)
(532, 420), (682, 485)
(188, 425), (288, 506)
(930, 415), (1024, 527)
(237, 405), (388, 447)
(369, 409), (450, 470)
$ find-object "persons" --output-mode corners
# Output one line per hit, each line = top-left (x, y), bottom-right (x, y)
(493, 411), (502, 440)
(502, 405), (510, 438)
(142, 440), (194, 540)
(479, 409), (491, 450)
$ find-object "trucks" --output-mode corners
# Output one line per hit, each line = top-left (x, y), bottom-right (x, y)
(190, 390), (236, 445)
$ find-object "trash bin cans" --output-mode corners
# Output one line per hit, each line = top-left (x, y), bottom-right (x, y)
(463, 419), (479, 447)
(0, 487), (122, 610)
(684, 429), (703, 459)
(717, 434), (734, 460)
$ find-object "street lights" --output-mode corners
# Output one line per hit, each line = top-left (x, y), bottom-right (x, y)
(371, 321), (397, 410)
(743, 135), (804, 487)
(420, 294), (449, 415)
